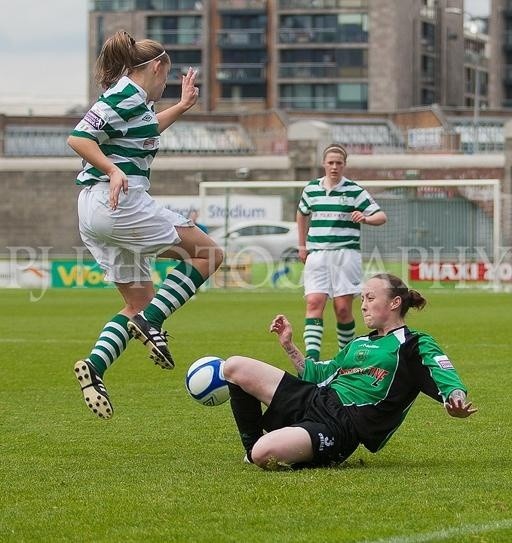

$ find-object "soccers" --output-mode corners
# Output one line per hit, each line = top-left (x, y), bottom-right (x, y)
(185, 356), (231, 407)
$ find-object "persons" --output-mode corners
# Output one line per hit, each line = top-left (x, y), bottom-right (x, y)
(295, 143), (390, 362)
(186, 208), (209, 235)
(64, 26), (225, 420)
(223, 272), (479, 470)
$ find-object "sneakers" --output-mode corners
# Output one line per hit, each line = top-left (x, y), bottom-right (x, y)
(126, 311), (176, 371)
(72, 360), (114, 420)
